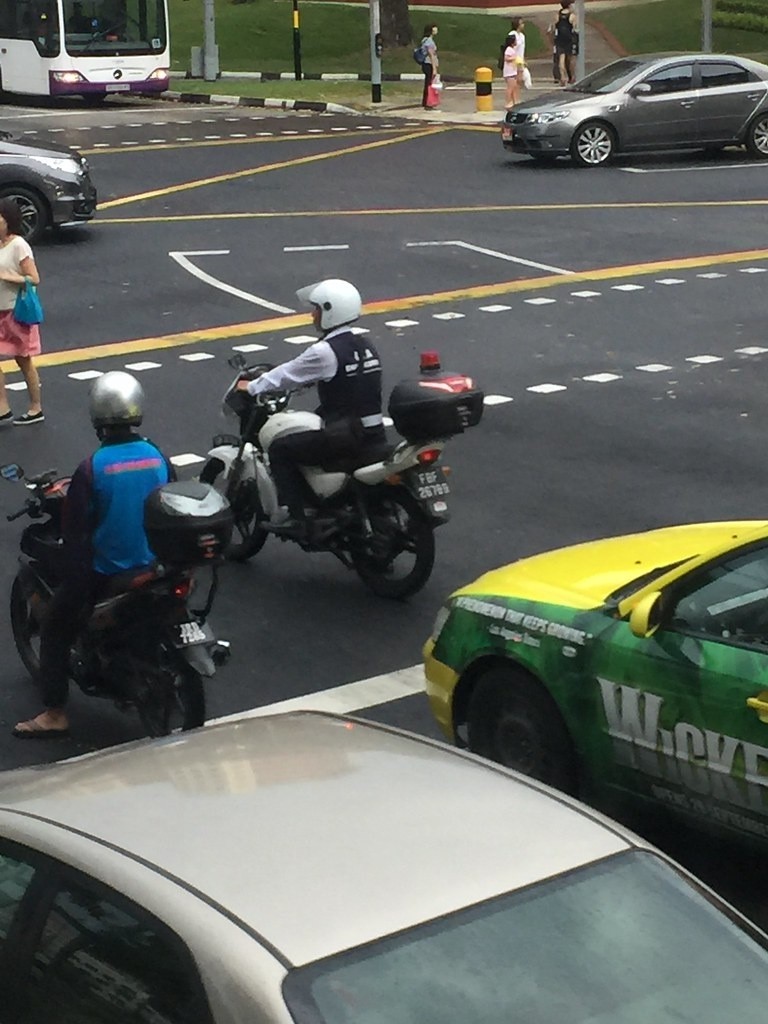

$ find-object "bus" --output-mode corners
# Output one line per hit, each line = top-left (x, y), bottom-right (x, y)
(0, 0), (171, 102)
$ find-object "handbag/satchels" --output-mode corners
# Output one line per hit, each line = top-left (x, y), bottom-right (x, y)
(432, 74), (443, 89)
(522, 67), (532, 90)
(568, 78), (575, 84)
(426, 84), (440, 108)
(12, 276), (45, 325)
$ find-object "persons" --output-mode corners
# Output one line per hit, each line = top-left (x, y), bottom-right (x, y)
(0, 200), (44, 425)
(421, 23), (438, 111)
(69, 4), (91, 33)
(551, 0), (578, 85)
(503, 17), (526, 108)
(10, 371), (178, 739)
(235, 279), (387, 533)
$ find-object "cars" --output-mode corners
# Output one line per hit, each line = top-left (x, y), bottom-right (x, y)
(500, 50), (768, 168)
(420, 520), (768, 859)
(0, 131), (98, 247)
(0, 711), (768, 1024)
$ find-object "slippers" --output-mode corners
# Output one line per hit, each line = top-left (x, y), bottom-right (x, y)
(12, 718), (72, 739)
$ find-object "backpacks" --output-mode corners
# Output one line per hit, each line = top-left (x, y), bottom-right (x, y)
(498, 43), (507, 70)
(412, 47), (426, 66)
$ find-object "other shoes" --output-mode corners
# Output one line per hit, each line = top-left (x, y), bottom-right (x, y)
(0, 410), (15, 423)
(257, 516), (310, 537)
(554, 78), (559, 83)
(423, 108), (438, 112)
(13, 410), (45, 425)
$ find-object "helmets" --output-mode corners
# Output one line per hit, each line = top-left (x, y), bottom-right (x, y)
(308, 278), (362, 329)
(88, 370), (145, 427)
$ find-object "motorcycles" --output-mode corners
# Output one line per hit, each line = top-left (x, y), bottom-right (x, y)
(0, 461), (233, 739)
(199, 350), (484, 600)
